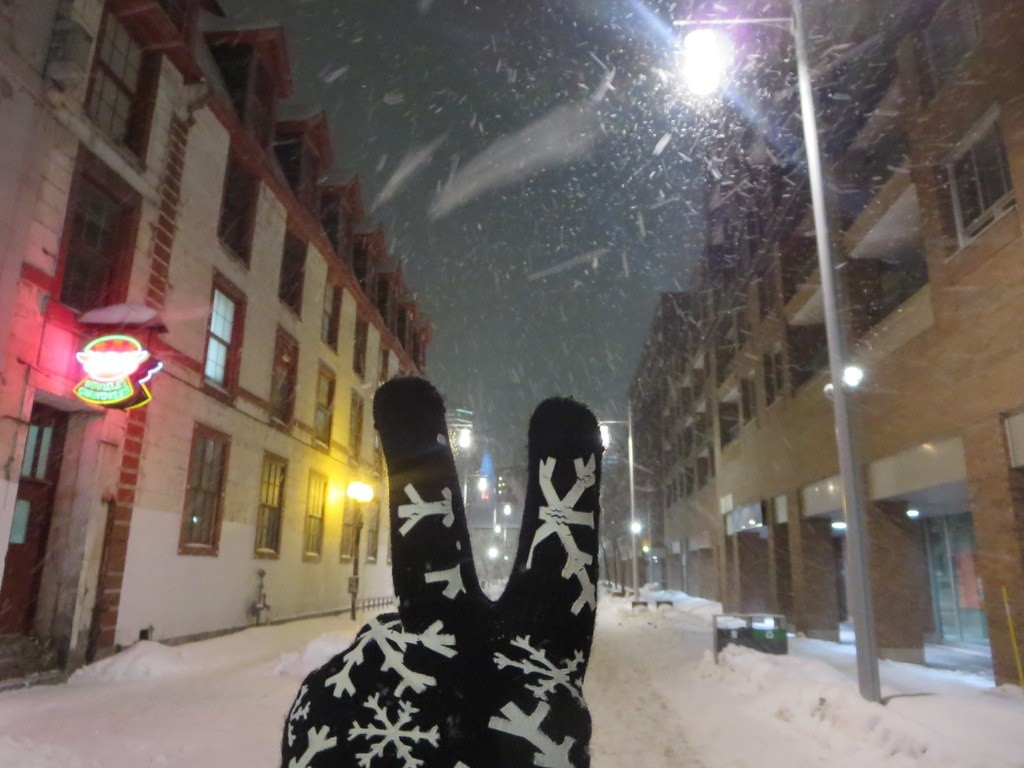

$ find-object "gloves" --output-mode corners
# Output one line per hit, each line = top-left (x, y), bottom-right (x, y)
(279, 376), (605, 768)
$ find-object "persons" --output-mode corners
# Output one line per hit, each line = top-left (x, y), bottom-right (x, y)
(283, 377), (603, 768)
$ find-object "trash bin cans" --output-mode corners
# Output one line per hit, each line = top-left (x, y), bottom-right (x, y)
(713, 612), (788, 664)
